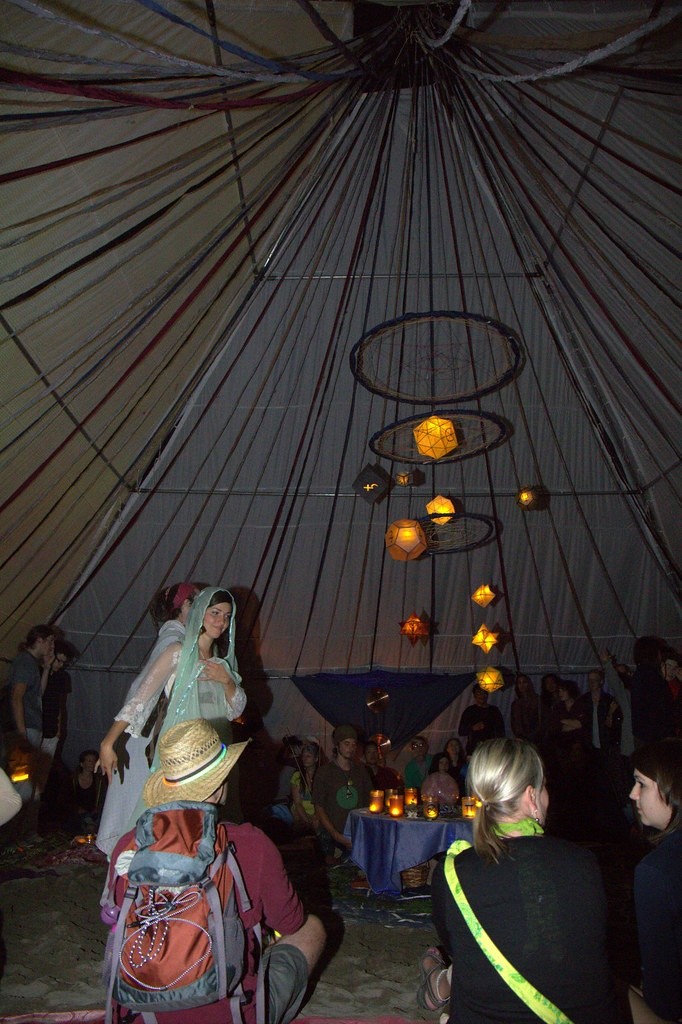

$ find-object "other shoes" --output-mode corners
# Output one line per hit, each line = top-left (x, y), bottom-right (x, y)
(352, 878), (371, 890)
(325, 855), (341, 865)
(15, 836), (34, 848)
(22, 832), (45, 842)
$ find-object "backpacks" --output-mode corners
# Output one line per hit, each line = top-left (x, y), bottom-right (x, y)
(105, 800), (265, 1011)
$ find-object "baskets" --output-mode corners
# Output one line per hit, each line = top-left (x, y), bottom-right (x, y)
(400, 864), (430, 888)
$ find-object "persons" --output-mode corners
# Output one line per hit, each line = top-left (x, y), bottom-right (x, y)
(278, 651), (681, 862)
(98, 717), (327, 1023)
(1, 623), (108, 835)
(94, 581), (248, 865)
(417, 737), (623, 1024)
(627, 738), (682, 1024)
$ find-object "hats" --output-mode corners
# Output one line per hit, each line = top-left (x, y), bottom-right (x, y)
(143, 718), (252, 808)
(332, 725), (357, 744)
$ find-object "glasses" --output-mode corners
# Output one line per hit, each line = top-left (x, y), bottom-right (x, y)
(413, 742), (426, 749)
(56, 657), (70, 667)
(475, 690), (488, 696)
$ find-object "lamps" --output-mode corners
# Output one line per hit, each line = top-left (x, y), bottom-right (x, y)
(352, 413), (541, 693)
(370, 787), (478, 820)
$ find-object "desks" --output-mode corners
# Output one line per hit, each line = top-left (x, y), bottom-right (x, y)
(353, 805), (473, 888)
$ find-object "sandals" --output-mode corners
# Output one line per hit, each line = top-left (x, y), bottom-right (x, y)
(417, 946), (451, 1010)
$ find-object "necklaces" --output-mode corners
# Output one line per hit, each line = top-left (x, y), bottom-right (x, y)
(307, 771), (313, 784)
(341, 770), (353, 799)
